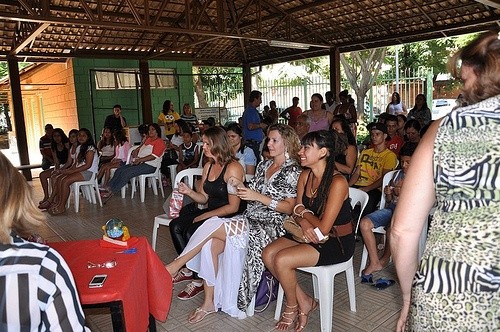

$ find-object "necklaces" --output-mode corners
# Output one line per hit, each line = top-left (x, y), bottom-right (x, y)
(310, 173), (318, 195)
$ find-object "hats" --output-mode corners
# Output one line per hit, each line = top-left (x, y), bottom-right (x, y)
(371, 123), (391, 142)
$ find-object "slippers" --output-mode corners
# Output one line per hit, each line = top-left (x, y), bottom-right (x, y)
(188, 307), (216, 324)
(361, 272), (373, 284)
(371, 278), (396, 291)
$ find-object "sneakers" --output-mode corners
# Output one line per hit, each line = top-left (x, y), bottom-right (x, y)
(172, 271), (192, 284)
(178, 282), (205, 300)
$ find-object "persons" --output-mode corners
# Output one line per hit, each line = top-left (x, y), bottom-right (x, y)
(0, 151), (91, 332)
(389, 31), (500, 332)
(37, 90), (434, 332)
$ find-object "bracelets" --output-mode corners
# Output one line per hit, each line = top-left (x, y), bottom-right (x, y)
(267, 199), (278, 210)
(302, 209), (315, 218)
(293, 203), (305, 216)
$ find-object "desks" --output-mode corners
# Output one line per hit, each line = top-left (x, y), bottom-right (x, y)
(40, 237), (173, 332)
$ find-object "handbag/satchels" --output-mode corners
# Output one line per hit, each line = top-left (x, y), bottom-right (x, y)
(254, 271), (279, 313)
(280, 215), (329, 243)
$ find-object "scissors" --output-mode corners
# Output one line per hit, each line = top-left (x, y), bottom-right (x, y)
(114, 248), (137, 254)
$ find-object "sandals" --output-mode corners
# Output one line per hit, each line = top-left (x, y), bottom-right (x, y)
(276, 303), (299, 331)
(295, 298), (319, 332)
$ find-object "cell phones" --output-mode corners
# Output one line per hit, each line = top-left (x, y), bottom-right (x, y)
(89, 275), (107, 286)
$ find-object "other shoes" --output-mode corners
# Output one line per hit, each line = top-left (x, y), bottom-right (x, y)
(38, 197), (66, 214)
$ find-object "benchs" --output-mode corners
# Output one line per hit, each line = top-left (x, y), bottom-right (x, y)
(13, 163), (49, 181)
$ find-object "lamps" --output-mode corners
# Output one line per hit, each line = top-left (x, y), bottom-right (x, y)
(267, 39), (310, 49)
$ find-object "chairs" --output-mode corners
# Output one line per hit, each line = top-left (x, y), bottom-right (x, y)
(47, 128), (435, 332)
